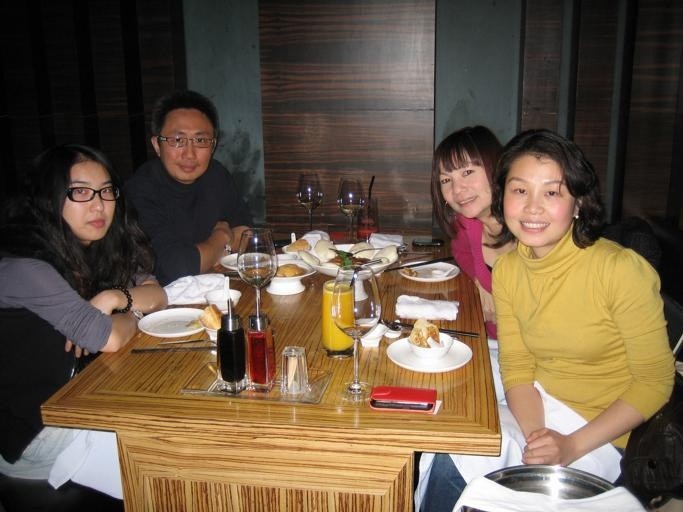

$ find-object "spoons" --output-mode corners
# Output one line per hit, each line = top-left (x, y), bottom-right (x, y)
(328, 248), (383, 257)
(382, 317), (481, 338)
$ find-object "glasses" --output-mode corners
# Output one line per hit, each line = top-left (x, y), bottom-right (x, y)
(67, 186), (120, 202)
(158, 137), (216, 148)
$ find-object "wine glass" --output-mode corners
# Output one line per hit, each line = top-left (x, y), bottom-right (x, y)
(333, 266), (382, 403)
(268, 254), (312, 296)
(336, 175), (365, 244)
(240, 227), (278, 337)
(295, 173), (326, 234)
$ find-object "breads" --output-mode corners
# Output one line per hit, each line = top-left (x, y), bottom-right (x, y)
(286, 239), (309, 253)
(407, 317), (440, 348)
(201, 303), (224, 329)
(276, 264), (301, 277)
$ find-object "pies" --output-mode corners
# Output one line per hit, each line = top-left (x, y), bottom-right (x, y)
(299, 238), (397, 272)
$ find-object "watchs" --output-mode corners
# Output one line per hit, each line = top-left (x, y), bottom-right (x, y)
(130, 309), (143, 334)
(224, 244), (232, 255)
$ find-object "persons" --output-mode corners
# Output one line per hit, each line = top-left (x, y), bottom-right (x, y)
(0, 144), (167, 499)
(430, 126), (518, 338)
(121, 89), (252, 287)
(413, 128), (676, 512)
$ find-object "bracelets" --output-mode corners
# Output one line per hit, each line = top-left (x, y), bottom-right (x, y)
(111, 286), (132, 314)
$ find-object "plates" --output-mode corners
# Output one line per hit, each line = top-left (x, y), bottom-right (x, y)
(386, 338), (473, 374)
(316, 256), (390, 278)
(137, 307), (206, 339)
(386, 360), (474, 395)
(399, 278), (460, 296)
(397, 261), (460, 283)
(221, 251), (272, 269)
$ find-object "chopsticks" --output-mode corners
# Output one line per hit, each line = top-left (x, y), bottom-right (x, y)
(384, 256), (454, 273)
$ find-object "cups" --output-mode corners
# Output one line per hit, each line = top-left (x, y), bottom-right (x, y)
(407, 331), (451, 362)
(357, 197), (378, 242)
(323, 281), (356, 359)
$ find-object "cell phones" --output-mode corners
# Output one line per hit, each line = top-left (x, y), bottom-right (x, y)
(368, 386), (437, 413)
(255, 239), (298, 252)
(413, 237), (444, 246)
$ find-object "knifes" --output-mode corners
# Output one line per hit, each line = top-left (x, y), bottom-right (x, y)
(130, 347), (218, 354)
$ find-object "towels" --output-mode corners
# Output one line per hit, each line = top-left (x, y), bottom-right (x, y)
(365, 227), (404, 249)
(162, 273), (229, 306)
(395, 295), (459, 322)
(300, 229), (326, 248)
(453, 473), (647, 512)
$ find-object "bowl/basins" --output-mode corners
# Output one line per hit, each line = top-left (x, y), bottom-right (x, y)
(200, 315), (220, 341)
(206, 290), (241, 313)
(460, 463), (616, 512)
(281, 245), (303, 259)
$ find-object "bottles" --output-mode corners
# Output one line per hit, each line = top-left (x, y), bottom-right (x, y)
(217, 300), (245, 390)
(280, 344), (310, 395)
(247, 295), (275, 391)
